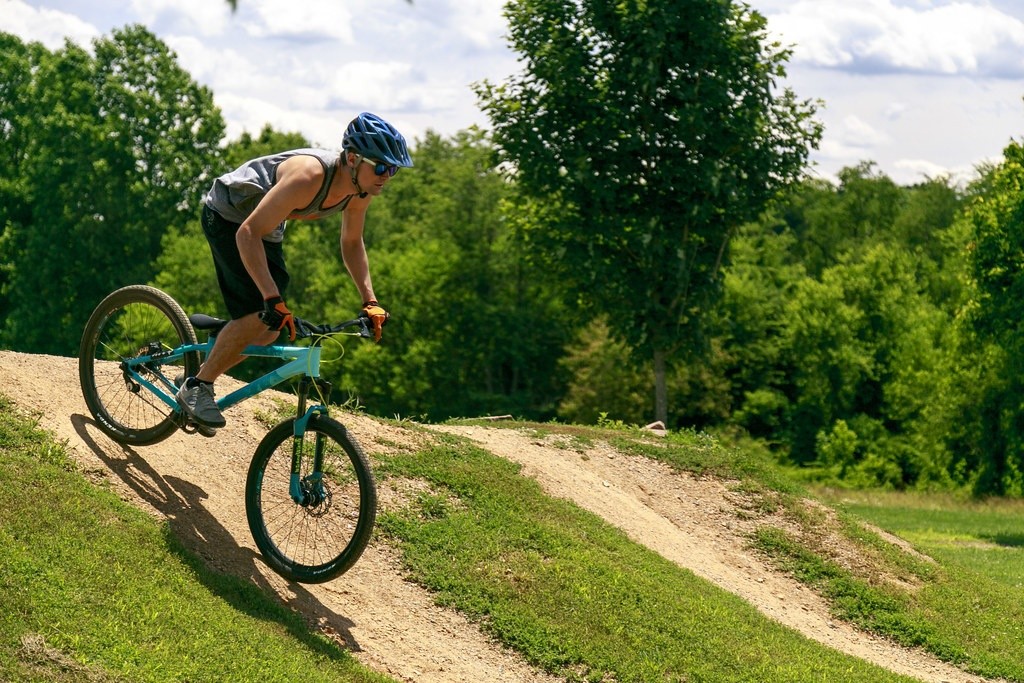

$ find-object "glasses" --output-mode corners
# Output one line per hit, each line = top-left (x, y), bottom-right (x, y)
(356, 153), (400, 177)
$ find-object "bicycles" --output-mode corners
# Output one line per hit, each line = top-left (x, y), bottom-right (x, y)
(77, 282), (377, 585)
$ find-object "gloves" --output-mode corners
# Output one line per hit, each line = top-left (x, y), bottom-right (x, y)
(362, 300), (387, 343)
(261, 296), (296, 342)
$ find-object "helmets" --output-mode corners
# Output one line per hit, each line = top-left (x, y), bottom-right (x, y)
(342, 112), (414, 168)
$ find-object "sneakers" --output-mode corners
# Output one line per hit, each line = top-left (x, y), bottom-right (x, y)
(175, 377), (226, 428)
(174, 373), (216, 437)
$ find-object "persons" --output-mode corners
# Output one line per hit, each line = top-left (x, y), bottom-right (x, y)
(175, 112), (414, 438)
